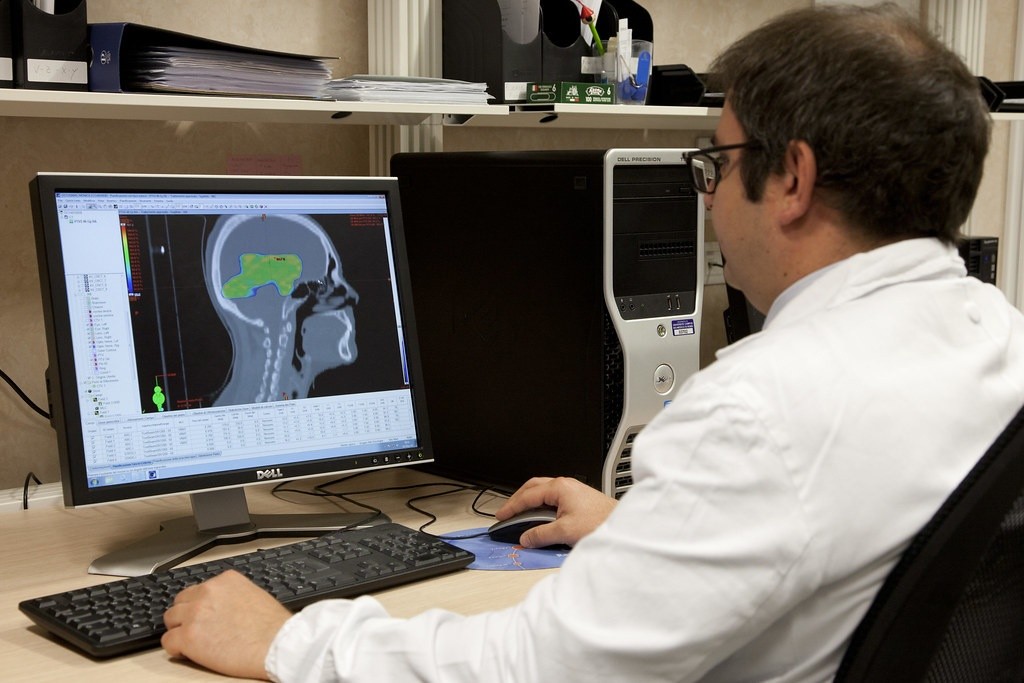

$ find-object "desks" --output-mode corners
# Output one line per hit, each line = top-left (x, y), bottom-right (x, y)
(0, 467), (559, 683)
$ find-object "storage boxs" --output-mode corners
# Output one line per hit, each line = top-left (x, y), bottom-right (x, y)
(527, 82), (618, 103)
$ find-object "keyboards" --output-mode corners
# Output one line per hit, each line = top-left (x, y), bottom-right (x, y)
(17, 525), (476, 659)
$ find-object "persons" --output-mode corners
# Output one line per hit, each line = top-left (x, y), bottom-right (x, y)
(162, 4), (1024, 682)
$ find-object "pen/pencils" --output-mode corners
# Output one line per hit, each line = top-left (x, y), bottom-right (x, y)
(581, 6), (604, 55)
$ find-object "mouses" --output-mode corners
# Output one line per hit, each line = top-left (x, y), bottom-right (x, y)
(485, 505), (572, 552)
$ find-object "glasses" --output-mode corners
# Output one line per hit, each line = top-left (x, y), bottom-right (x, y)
(680, 140), (764, 194)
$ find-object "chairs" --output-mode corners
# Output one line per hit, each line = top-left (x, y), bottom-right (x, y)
(832, 405), (1024, 683)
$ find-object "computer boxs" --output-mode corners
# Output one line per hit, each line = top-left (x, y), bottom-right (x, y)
(391, 147), (706, 501)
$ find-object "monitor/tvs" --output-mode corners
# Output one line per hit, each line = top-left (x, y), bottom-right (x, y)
(28, 171), (436, 578)
(720, 252), (767, 345)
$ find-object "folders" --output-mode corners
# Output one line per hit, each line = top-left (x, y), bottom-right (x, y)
(89, 21), (341, 103)
